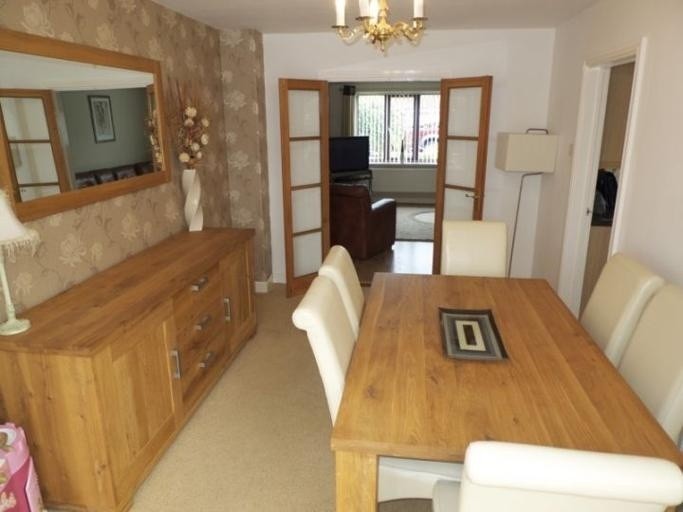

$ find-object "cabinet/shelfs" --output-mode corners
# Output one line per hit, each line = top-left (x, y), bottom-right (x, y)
(0, 227), (257, 512)
(330, 170), (372, 195)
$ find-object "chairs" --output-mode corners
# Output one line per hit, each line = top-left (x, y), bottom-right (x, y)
(580, 252), (666, 368)
(433, 441), (683, 511)
(291, 274), (463, 512)
(440, 220), (507, 277)
(329, 184), (396, 260)
(616, 283), (683, 445)
(318, 244), (364, 340)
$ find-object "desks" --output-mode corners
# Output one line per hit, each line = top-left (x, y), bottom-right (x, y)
(330, 272), (683, 511)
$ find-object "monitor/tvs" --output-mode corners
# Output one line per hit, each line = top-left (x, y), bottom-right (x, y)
(328, 135), (370, 173)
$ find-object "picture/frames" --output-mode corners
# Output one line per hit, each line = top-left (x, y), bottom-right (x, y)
(87, 94), (116, 143)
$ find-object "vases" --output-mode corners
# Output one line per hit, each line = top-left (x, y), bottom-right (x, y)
(182, 170), (203, 232)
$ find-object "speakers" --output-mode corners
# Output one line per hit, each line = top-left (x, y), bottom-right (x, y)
(344, 85), (355, 96)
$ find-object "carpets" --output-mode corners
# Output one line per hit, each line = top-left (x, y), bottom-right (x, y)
(394, 203), (436, 241)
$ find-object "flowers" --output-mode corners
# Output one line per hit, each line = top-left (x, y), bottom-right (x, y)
(177, 104), (210, 169)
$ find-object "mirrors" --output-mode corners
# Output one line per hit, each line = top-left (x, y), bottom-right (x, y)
(0, 27), (172, 222)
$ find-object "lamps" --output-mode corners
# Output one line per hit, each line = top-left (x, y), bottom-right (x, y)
(0, 191), (38, 335)
(331, 0), (428, 50)
(495, 129), (559, 279)
